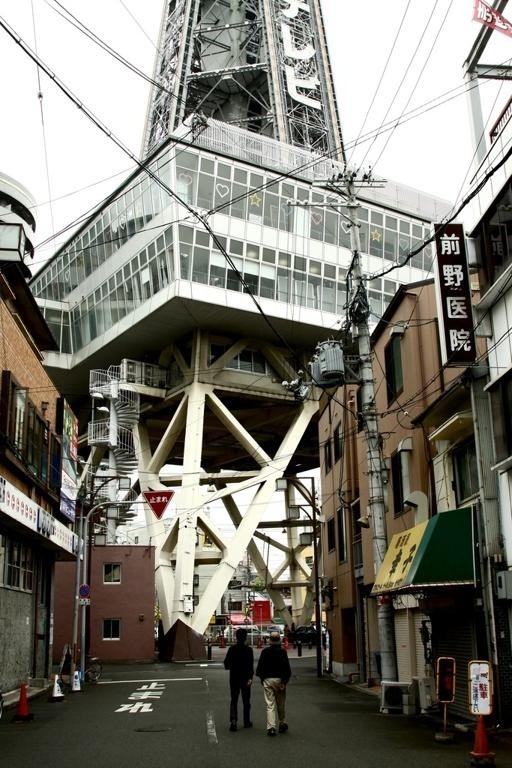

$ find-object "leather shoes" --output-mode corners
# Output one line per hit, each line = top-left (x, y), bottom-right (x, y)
(278, 722), (288, 733)
(267, 727), (276, 735)
(244, 721), (253, 728)
(229, 723), (237, 731)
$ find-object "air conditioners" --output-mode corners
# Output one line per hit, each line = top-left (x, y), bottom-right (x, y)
(380, 680), (411, 710)
(120, 357), (160, 388)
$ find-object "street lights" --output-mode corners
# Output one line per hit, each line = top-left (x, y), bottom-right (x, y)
(84, 472), (130, 679)
(275, 473), (326, 678)
(244, 593), (253, 627)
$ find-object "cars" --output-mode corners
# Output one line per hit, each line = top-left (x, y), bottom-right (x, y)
(204, 621), (328, 646)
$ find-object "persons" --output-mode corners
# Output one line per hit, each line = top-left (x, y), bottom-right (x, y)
(290, 622), (295, 632)
(283, 624), (288, 643)
(255, 631), (292, 736)
(223, 628), (254, 732)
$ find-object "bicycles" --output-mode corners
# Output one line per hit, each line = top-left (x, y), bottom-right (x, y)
(60, 647), (105, 686)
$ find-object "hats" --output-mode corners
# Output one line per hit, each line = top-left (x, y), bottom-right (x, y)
(268, 632), (281, 645)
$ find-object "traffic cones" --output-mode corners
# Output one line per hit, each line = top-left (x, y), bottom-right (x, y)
(48, 674), (66, 704)
(69, 667), (85, 694)
(469, 715), (498, 760)
(12, 682), (37, 724)
